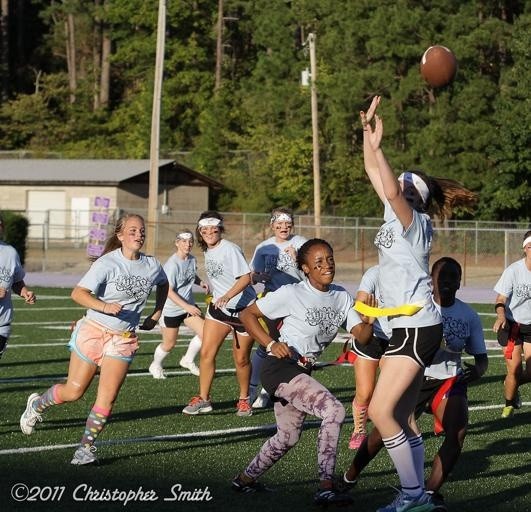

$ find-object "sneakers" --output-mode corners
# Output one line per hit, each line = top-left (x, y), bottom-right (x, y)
(20, 393), (47, 435)
(251, 388), (270, 408)
(180, 356), (200, 376)
(182, 397), (212, 414)
(501, 399), (517, 418)
(232, 475), (272, 496)
(314, 490), (353, 507)
(426, 490), (448, 512)
(149, 363), (167, 379)
(348, 431), (366, 450)
(236, 396), (252, 416)
(70, 447), (100, 466)
(312, 475), (356, 503)
(375, 491), (436, 512)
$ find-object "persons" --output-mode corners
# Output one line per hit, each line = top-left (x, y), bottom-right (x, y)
(492, 231), (531, 419)
(148, 95), (488, 511)
(19, 214), (169, 466)
(0, 214), (37, 358)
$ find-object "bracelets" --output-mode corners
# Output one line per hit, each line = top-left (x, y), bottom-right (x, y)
(102, 303), (106, 313)
(494, 303), (507, 313)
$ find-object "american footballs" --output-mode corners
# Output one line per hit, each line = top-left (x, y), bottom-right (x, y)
(420, 44), (456, 90)
